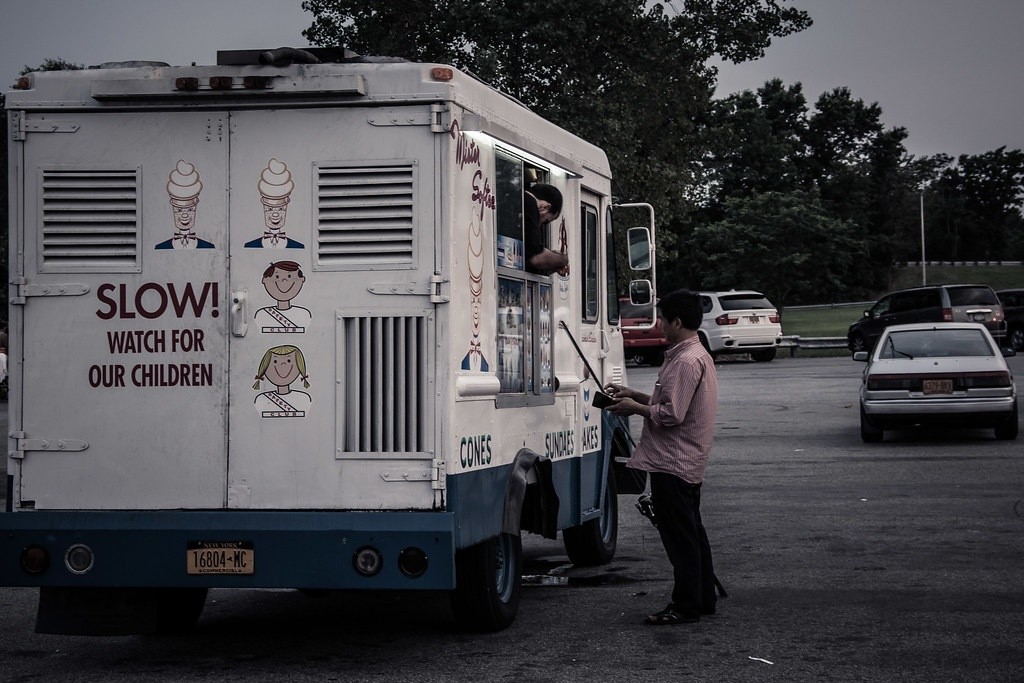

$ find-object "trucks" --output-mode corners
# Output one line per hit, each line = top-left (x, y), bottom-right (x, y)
(1, 47), (659, 642)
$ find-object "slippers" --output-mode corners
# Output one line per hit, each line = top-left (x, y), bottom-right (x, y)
(645, 604), (700, 625)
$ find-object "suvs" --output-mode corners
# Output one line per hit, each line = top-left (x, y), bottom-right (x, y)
(588, 295), (671, 368)
(695, 289), (783, 365)
(847, 283), (1008, 361)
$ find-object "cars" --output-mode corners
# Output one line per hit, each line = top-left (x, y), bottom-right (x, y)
(997, 287), (1024, 353)
(854, 320), (1020, 446)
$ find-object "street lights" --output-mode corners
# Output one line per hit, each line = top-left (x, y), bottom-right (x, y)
(917, 183), (928, 287)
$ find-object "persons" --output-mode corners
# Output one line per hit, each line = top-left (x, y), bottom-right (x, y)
(497, 183), (569, 274)
(604, 289), (717, 624)
